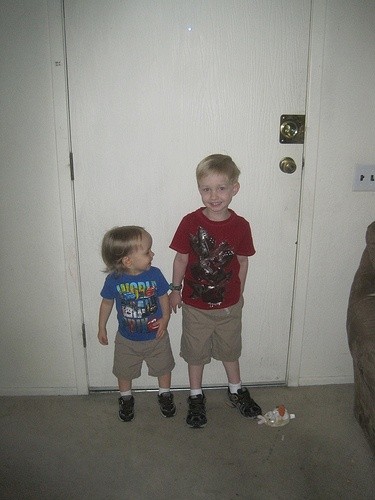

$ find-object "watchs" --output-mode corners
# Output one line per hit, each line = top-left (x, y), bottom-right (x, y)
(168, 282), (182, 290)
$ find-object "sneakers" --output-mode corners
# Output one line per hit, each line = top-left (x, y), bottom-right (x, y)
(186, 391), (207, 428)
(119, 395), (134, 420)
(158, 391), (176, 418)
(224, 386), (263, 418)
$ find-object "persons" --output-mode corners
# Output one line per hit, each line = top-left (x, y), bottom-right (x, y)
(168, 154), (264, 428)
(98, 226), (178, 423)
